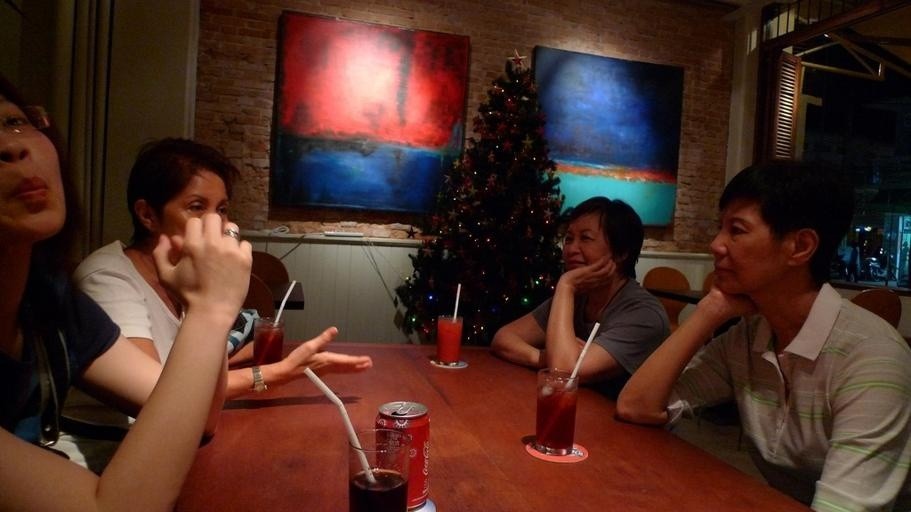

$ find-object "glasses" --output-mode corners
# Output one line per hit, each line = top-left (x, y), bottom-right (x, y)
(0, 104), (51, 136)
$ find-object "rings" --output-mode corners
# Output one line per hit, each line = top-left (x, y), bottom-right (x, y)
(223, 229), (240, 240)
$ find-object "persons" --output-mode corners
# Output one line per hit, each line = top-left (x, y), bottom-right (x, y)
(491, 195), (675, 395)
(0, 84), (254, 511)
(616, 156), (911, 512)
(53, 135), (372, 469)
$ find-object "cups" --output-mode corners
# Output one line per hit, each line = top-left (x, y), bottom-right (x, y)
(436, 315), (463, 367)
(533, 367), (580, 456)
(253, 316), (285, 366)
(347, 428), (411, 512)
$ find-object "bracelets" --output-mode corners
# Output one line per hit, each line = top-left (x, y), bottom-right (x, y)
(252, 364), (268, 396)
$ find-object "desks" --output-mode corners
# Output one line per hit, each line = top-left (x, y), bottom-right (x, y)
(176, 340), (817, 512)
(648, 284), (741, 335)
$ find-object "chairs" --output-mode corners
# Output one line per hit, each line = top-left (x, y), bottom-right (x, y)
(848, 288), (903, 333)
(643, 265), (693, 338)
(240, 249), (305, 318)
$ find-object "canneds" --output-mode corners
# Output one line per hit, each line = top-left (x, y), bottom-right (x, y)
(376, 401), (430, 511)
